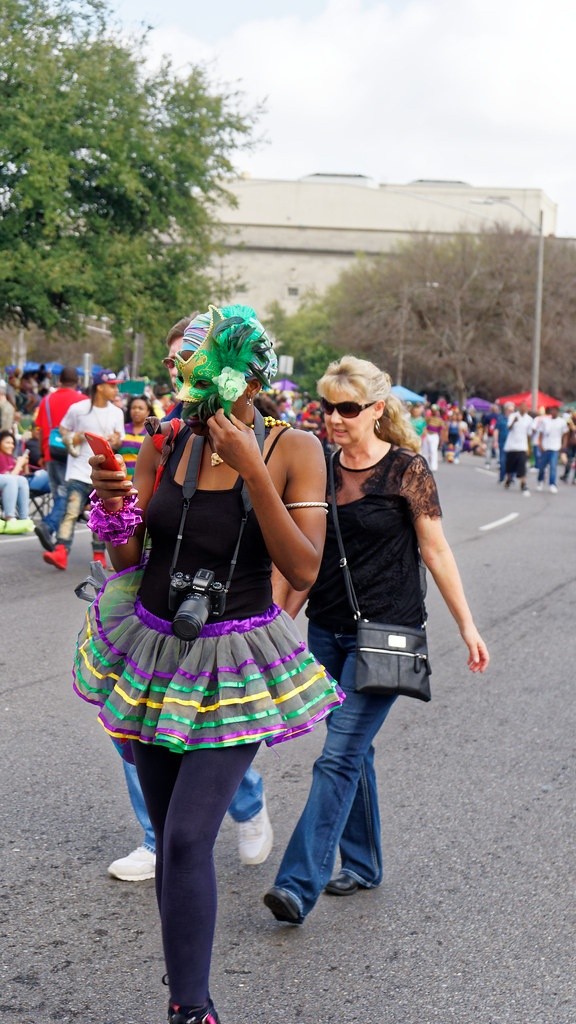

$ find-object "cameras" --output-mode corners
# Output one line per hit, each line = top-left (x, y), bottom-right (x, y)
(168, 568), (227, 642)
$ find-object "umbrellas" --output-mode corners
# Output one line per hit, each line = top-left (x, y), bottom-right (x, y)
(388, 385), (564, 410)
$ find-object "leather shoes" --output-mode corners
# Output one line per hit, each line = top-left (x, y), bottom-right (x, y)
(325, 873), (359, 895)
(264, 888), (304, 924)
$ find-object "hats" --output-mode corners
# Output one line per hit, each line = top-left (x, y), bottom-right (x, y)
(94, 370), (124, 385)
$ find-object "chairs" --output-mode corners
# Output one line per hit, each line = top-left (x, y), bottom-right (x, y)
(29, 487), (52, 520)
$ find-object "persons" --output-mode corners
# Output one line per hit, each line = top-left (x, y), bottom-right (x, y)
(72, 301), (327, 1024)
(119, 394), (159, 480)
(277, 398), (289, 423)
(107, 312), (273, 881)
(536, 406), (570, 493)
(0, 364), (124, 572)
(409, 399), (486, 470)
(262, 356), (490, 925)
(559, 413), (576, 484)
(502, 401), (534, 491)
(151, 382), (173, 421)
(493, 401), (516, 483)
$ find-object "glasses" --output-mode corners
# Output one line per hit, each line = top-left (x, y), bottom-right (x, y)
(321, 397), (376, 418)
(163, 358), (176, 369)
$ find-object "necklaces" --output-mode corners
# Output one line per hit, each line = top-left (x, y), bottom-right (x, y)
(208, 416), (292, 467)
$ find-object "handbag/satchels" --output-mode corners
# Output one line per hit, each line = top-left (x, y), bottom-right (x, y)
(49, 428), (68, 457)
(354, 619), (432, 702)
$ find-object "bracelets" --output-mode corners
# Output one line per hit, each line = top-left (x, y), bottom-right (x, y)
(87, 495), (143, 547)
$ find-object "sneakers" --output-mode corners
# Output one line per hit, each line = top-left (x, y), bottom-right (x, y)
(237, 791), (274, 865)
(108, 846), (156, 882)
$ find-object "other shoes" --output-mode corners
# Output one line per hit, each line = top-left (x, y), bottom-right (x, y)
(521, 487), (529, 497)
(537, 480), (544, 491)
(505, 479), (512, 488)
(44, 544), (68, 570)
(34, 522), (55, 552)
(168, 999), (220, 1024)
(94, 550), (107, 569)
(549, 484), (558, 493)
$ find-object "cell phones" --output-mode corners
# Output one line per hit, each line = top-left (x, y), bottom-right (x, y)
(84, 431), (131, 492)
(23, 450), (30, 457)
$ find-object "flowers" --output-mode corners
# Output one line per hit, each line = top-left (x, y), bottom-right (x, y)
(211, 366), (249, 402)
